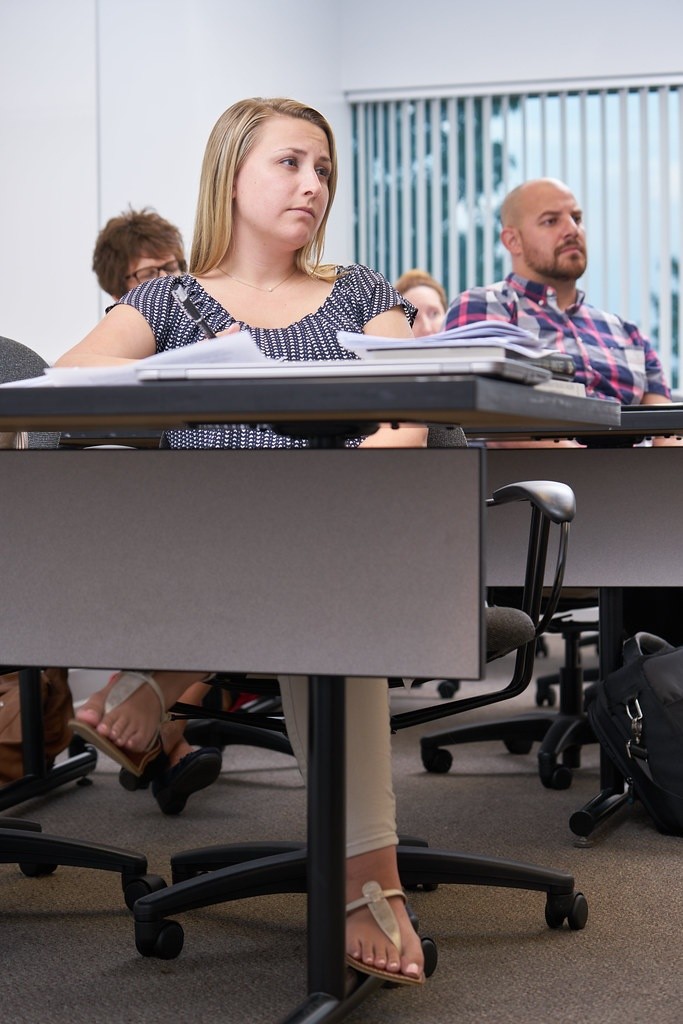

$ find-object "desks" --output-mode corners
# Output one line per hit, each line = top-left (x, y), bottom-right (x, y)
(1, 375), (623, 1024)
(467, 409), (683, 838)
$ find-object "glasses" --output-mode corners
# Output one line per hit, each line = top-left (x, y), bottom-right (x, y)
(125, 259), (179, 285)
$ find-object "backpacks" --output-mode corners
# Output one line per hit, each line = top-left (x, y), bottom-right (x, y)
(583, 631), (683, 837)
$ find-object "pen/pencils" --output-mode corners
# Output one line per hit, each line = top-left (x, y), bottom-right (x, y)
(168, 278), (217, 340)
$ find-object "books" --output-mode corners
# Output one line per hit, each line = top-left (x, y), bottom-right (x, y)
(367, 346), (586, 395)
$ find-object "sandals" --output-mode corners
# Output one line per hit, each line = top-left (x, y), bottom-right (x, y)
(344, 880), (429, 989)
(67, 671), (166, 778)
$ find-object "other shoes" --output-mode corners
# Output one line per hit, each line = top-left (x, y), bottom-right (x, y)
(152, 747), (224, 818)
(118, 756), (153, 792)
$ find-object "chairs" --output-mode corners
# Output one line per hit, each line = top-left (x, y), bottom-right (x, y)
(0, 427), (598, 978)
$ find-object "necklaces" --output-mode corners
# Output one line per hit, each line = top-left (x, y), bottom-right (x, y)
(218, 267), (298, 291)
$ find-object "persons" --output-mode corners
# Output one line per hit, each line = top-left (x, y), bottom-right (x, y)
(393, 270), (445, 335)
(441, 176), (683, 650)
(94, 212), (221, 809)
(53, 98), (427, 984)
